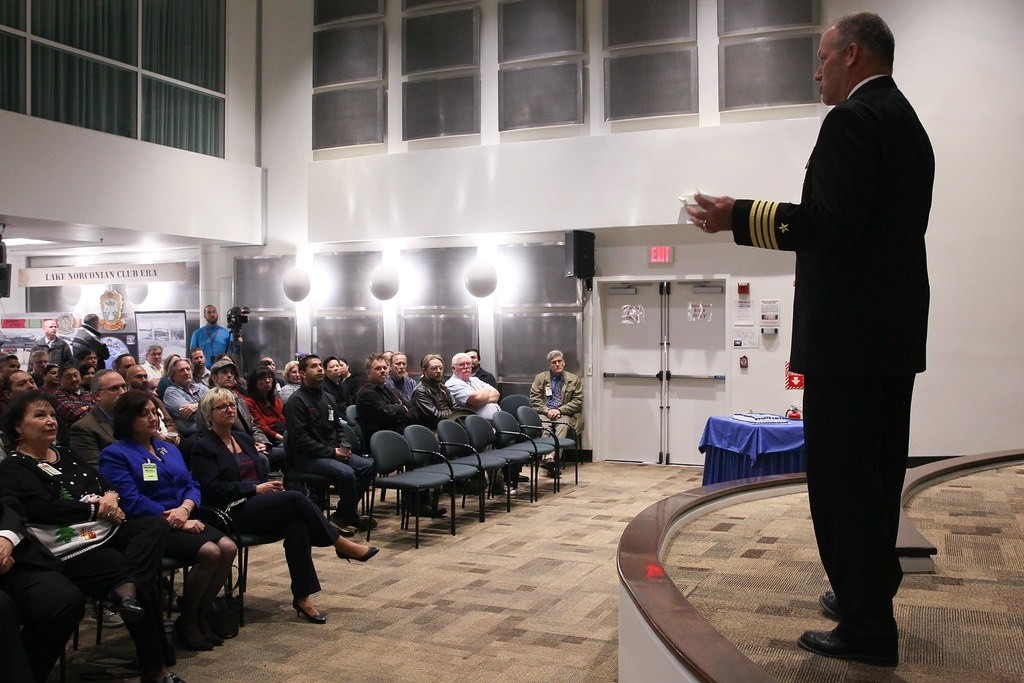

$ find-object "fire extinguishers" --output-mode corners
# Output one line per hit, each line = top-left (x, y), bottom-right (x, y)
(785, 404), (802, 419)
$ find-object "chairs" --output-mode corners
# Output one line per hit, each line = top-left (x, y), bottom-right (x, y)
(437, 421), (511, 524)
(498, 393), (533, 418)
(345, 405), (407, 515)
(491, 411), (559, 503)
(283, 427), (336, 518)
(395, 425), (482, 536)
(465, 415), (534, 512)
(57, 497), (285, 682)
(366, 430), (453, 550)
(517, 407), (579, 494)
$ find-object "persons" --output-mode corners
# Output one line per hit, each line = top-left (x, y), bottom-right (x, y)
(0, 314), (380, 683)
(189, 305), (243, 371)
(356, 349), (529, 517)
(530, 350), (583, 478)
(683, 12), (936, 666)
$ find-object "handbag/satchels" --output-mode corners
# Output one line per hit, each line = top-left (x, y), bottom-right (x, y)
(176, 565), (241, 639)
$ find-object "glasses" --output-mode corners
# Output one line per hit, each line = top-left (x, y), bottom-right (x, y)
(97, 384), (127, 392)
(210, 401), (238, 411)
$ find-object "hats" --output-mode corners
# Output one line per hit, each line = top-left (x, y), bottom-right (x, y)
(210, 360), (239, 381)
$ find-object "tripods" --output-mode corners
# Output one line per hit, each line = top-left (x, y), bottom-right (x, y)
(227, 329), (248, 377)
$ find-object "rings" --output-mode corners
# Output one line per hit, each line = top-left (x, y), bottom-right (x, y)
(108, 512), (113, 518)
(174, 524), (178, 527)
(703, 220), (709, 232)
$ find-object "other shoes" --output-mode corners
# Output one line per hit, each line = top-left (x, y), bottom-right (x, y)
(546, 466), (562, 477)
(161, 591), (183, 612)
(331, 514), (378, 530)
(518, 475), (529, 482)
(411, 504), (447, 517)
(90, 610), (124, 626)
(539, 458), (556, 469)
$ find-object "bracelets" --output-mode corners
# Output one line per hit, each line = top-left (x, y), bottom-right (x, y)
(104, 490), (120, 504)
(181, 506), (190, 516)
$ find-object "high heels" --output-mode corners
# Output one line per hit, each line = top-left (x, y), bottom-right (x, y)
(336, 547), (380, 563)
(173, 616), (214, 650)
(292, 600), (326, 624)
(206, 633), (223, 645)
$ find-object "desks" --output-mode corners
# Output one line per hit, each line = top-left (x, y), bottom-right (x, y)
(698, 413), (806, 487)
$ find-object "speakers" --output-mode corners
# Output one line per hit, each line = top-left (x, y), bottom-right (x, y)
(564, 230), (595, 278)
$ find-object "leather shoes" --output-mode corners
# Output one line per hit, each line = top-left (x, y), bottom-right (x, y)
(798, 630), (898, 667)
(140, 671), (186, 683)
(100, 590), (152, 617)
(820, 591), (841, 617)
